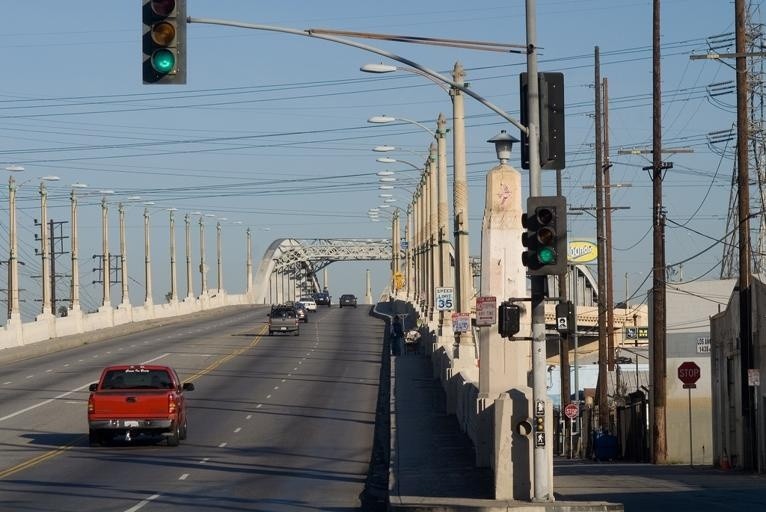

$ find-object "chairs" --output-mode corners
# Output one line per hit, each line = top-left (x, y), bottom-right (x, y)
(113, 375), (126, 388)
(150, 375), (161, 387)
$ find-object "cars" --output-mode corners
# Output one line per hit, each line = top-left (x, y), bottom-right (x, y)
(88, 363), (195, 445)
(340, 294), (357, 307)
(269, 292), (332, 334)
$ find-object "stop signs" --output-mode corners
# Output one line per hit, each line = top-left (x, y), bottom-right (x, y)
(564, 405), (578, 417)
(677, 363), (701, 384)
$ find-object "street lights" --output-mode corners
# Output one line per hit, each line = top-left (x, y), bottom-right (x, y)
(3, 164), (152, 315)
(145, 207), (271, 305)
(360, 62), (475, 343)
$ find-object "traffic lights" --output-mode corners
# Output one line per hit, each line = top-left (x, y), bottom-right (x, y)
(497, 306), (519, 336)
(141, 1), (188, 84)
(557, 301), (576, 334)
(521, 196), (567, 277)
